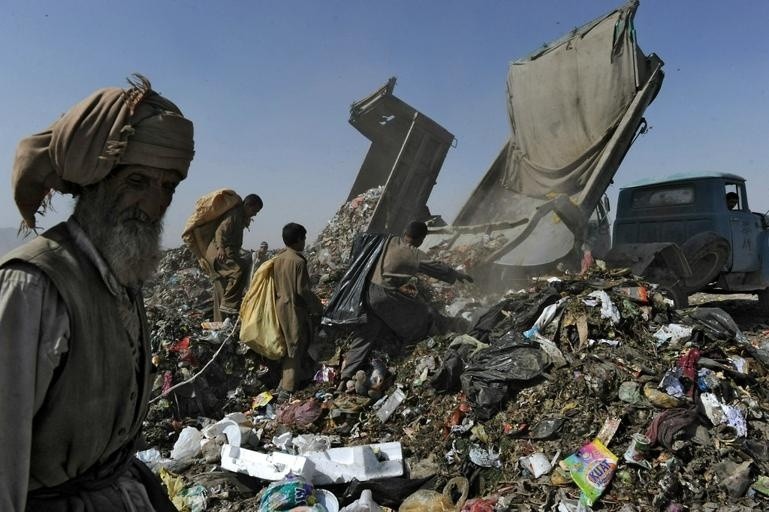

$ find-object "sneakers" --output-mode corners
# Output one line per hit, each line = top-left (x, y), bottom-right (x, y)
(277, 391), (290, 402)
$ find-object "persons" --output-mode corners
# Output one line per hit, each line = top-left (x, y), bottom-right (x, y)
(331, 221), (474, 398)
(204, 194), (264, 318)
(254, 223), (324, 402)
(726, 191), (739, 210)
(0, 73), (197, 511)
(248, 240), (276, 279)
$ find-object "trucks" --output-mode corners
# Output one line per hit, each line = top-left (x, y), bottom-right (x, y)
(442, 2), (769, 310)
(307, 76), (612, 284)
